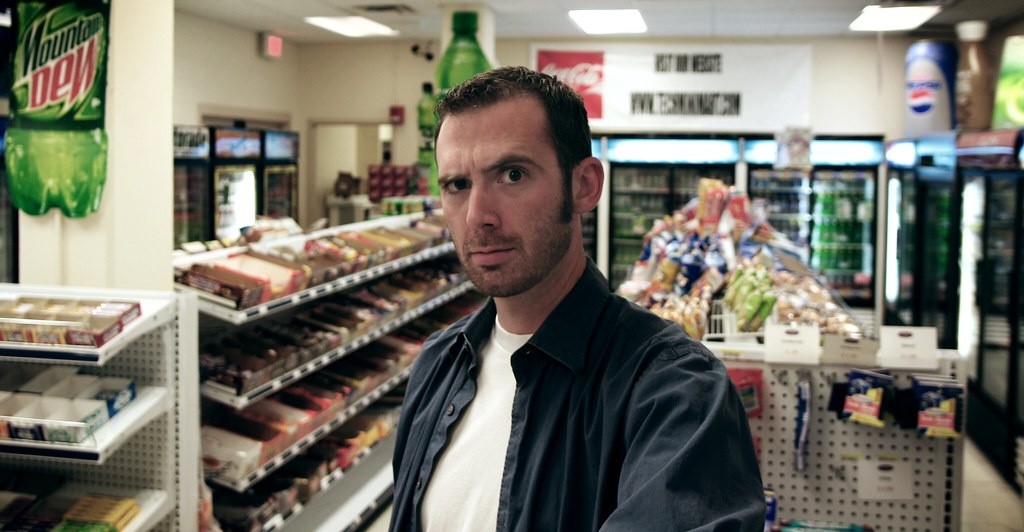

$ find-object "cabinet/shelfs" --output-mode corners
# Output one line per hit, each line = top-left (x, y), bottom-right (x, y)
(0, 283), (199, 532)
(174, 208), (475, 532)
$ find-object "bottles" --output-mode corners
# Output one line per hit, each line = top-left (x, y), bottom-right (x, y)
(416, 83), (438, 168)
(4, 1), (108, 218)
(431, 10), (491, 196)
(953, 22), (994, 131)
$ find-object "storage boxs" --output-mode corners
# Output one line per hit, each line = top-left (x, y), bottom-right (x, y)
(175, 210), (489, 532)
(0, 294), (141, 444)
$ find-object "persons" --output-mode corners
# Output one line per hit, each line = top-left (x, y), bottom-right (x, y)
(388, 66), (766, 531)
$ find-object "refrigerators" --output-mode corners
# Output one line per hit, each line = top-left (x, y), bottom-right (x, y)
(879, 132), (1024, 507)
(172, 125), (299, 252)
(584, 132), (882, 314)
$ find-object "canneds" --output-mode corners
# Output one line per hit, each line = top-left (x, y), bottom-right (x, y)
(901, 37), (953, 135)
